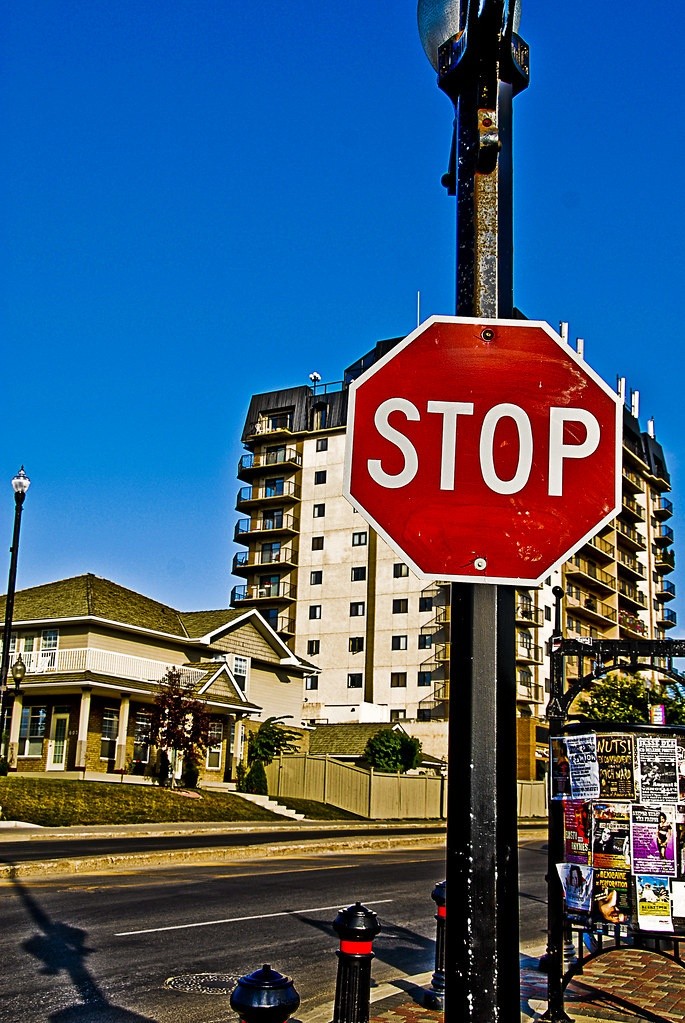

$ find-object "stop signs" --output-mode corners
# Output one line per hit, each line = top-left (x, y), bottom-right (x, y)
(342, 314), (625, 587)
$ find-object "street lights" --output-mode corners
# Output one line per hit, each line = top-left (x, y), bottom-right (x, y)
(0, 466), (31, 721)
